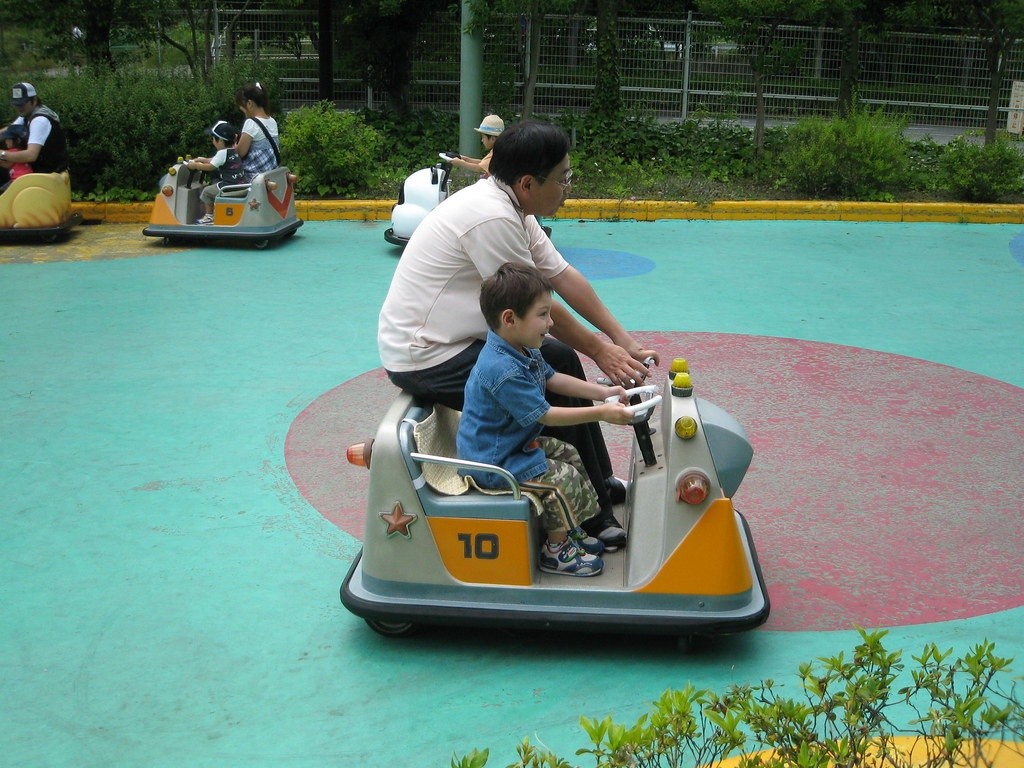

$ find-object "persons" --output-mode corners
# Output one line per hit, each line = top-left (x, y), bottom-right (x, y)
(451, 114), (505, 178)
(186, 123), (247, 225)
(234, 80), (281, 186)
(0, 82), (67, 195)
(455, 260), (638, 576)
(378, 117), (662, 544)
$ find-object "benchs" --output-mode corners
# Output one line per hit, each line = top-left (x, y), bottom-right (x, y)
(400, 407), (531, 520)
(215, 184), (251, 203)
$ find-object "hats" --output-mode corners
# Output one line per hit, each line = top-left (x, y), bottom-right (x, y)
(473, 115), (504, 137)
(204, 121), (235, 141)
(10, 82), (36, 105)
(1, 125), (28, 141)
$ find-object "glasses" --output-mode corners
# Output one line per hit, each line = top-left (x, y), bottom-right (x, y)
(534, 169), (574, 192)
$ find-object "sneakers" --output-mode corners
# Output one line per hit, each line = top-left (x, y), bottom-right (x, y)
(539, 536), (605, 577)
(582, 513), (626, 552)
(569, 526), (604, 556)
(604, 475), (626, 504)
(192, 214), (213, 225)
